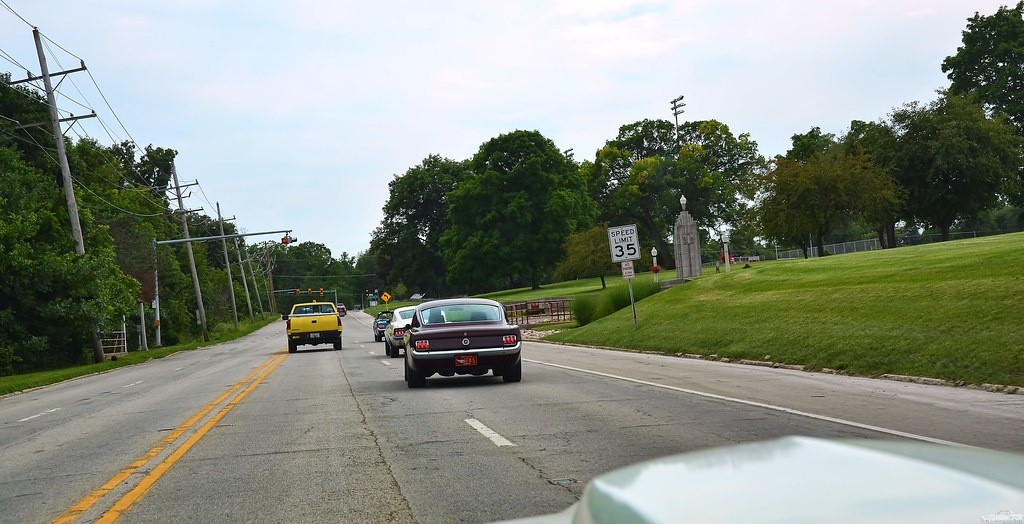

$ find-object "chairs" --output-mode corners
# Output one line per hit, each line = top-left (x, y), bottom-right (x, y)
(428, 309), (445, 324)
(471, 310), (490, 321)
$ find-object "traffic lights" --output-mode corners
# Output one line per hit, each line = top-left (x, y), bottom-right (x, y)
(365, 288), (369, 297)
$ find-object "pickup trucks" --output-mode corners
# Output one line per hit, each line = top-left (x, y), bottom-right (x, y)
(282, 299), (345, 353)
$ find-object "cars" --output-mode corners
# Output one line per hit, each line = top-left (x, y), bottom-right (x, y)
(487, 433), (1024, 524)
(337, 303), (347, 315)
(373, 298), (522, 388)
